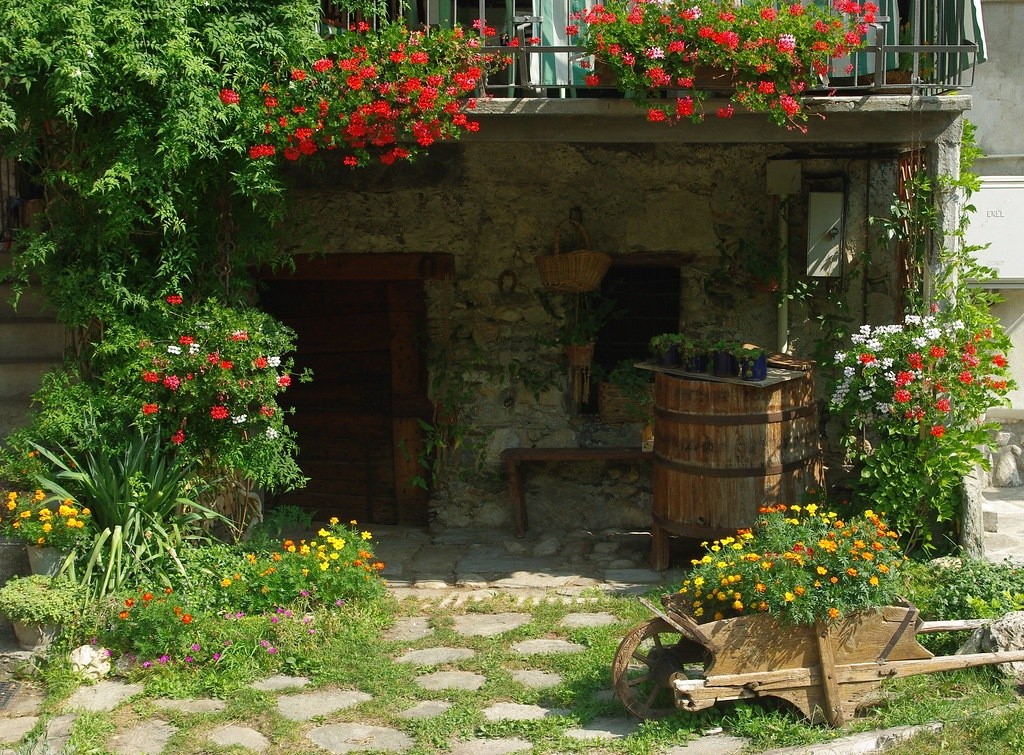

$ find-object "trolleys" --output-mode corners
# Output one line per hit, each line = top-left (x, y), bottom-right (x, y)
(611, 591), (1024, 729)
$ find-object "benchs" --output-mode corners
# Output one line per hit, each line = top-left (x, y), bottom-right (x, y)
(500, 447), (652, 539)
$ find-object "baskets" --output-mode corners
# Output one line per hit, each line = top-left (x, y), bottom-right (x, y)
(535, 218), (613, 293)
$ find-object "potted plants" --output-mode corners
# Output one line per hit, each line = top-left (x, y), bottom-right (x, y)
(677, 339), (711, 373)
(536, 284), (630, 366)
(0, 573), (80, 651)
(708, 337), (740, 378)
(588, 359), (655, 424)
(648, 333), (685, 367)
(736, 347), (770, 381)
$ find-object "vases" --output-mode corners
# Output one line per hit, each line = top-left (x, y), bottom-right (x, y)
(25, 543), (63, 577)
(594, 58), (759, 89)
(868, 69), (913, 94)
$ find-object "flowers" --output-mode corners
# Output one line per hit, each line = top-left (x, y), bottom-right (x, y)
(897, 17), (939, 79)
(216, 13), (542, 170)
(565, 0), (879, 137)
(674, 503), (913, 627)
(0, 488), (93, 551)
